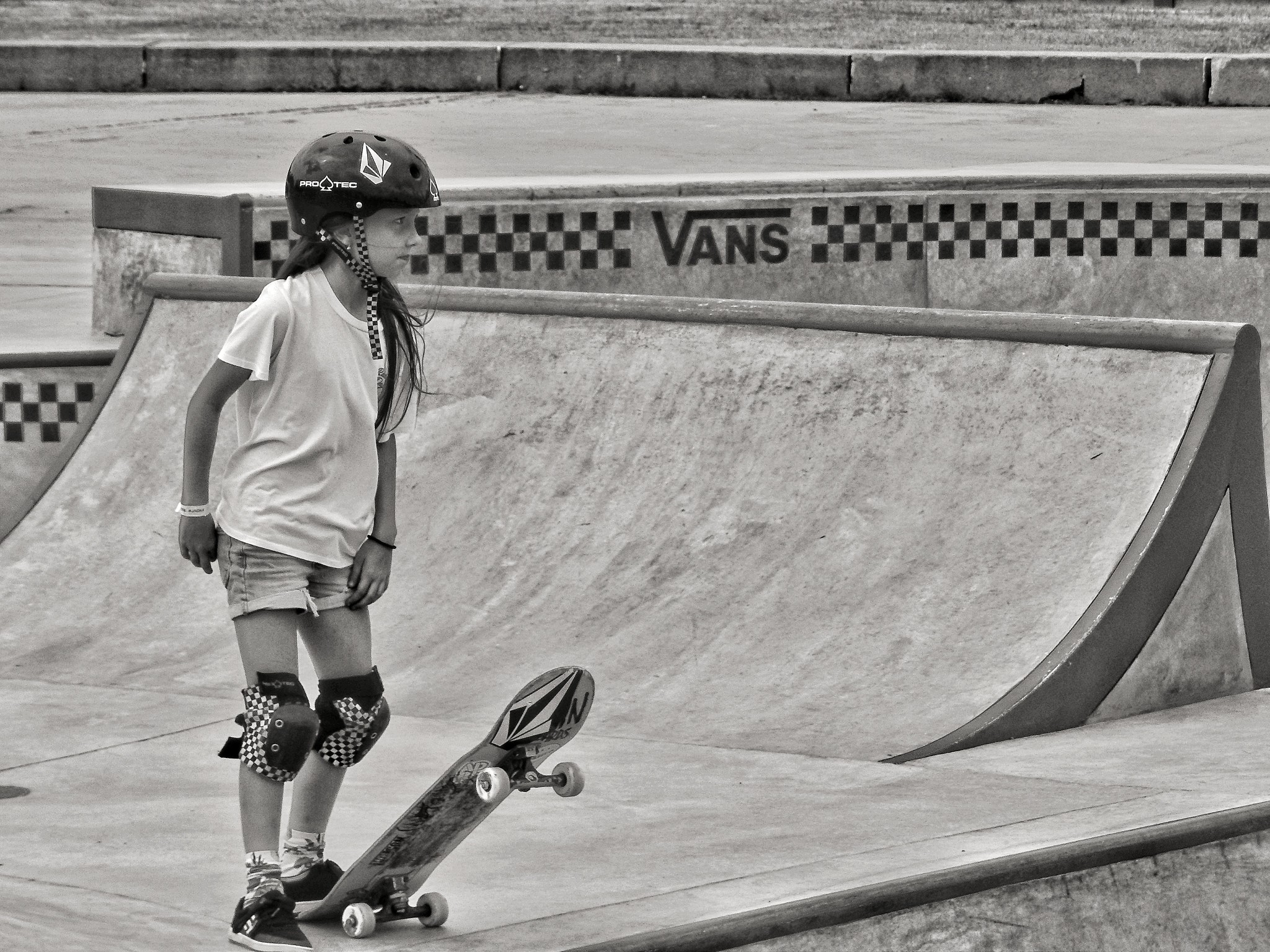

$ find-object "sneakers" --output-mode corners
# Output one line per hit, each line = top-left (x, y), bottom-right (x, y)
(283, 857), (344, 907)
(228, 891), (315, 952)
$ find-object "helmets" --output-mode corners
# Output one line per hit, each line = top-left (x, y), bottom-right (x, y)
(281, 133), (443, 239)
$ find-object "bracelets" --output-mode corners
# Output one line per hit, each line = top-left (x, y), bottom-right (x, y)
(176, 503), (211, 517)
(367, 535), (396, 549)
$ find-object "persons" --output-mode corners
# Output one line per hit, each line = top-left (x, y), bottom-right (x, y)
(178, 133), (441, 952)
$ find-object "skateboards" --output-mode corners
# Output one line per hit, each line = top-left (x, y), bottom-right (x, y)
(290, 665), (595, 939)
